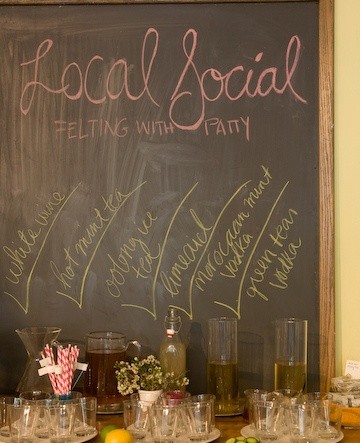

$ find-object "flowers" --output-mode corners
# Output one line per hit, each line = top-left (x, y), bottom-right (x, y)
(113, 354), (191, 397)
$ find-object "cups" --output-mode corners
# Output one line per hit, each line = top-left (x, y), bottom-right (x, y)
(274, 316), (307, 395)
(207, 317), (239, 413)
(85, 331), (141, 411)
(0, 390), (97, 443)
(243, 388), (342, 443)
(122, 390), (216, 443)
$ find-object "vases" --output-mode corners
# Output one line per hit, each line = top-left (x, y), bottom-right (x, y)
(138, 388), (164, 403)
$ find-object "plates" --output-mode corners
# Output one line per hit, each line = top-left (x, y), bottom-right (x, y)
(240, 419), (344, 443)
(0, 417), (98, 443)
(125, 415), (220, 443)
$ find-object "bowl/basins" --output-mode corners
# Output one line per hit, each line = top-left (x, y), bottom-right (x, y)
(326, 402), (360, 427)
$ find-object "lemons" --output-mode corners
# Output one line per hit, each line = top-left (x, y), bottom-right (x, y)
(100, 425), (133, 443)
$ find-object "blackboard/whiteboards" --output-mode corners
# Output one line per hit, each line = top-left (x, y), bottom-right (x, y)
(1, 0), (333, 396)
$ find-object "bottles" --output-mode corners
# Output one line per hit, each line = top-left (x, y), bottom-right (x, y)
(15, 326), (62, 395)
(159, 308), (187, 392)
